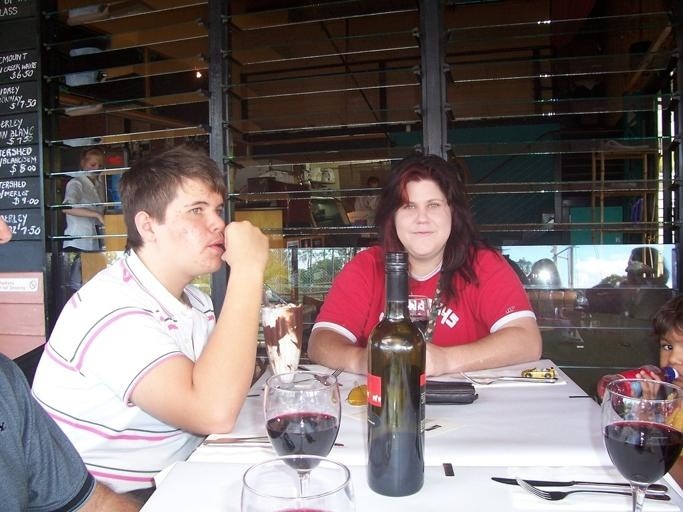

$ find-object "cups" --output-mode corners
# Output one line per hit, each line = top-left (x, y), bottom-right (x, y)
(522, 288), (591, 327)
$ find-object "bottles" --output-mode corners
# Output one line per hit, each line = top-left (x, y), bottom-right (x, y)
(364, 249), (426, 497)
(598, 364), (680, 407)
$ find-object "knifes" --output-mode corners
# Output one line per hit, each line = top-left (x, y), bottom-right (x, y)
(295, 364), (331, 387)
(491, 476), (669, 494)
(214, 434), (345, 447)
(450, 374), (558, 381)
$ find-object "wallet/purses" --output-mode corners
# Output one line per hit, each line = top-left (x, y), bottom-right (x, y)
(424, 381), (476, 402)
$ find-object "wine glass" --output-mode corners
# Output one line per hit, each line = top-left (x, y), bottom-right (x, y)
(601, 379), (682, 512)
(263, 369), (342, 498)
(259, 297), (302, 404)
(239, 453), (356, 512)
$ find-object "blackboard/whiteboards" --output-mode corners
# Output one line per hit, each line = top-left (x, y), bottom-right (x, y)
(0, 0), (45, 272)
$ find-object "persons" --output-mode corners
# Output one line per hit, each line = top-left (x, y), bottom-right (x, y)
(307, 150), (542, 380)
(353, 175), (382, 223)
(631, 292), (682, 489)
(30, 142), (270, 503)
(528, 257), (577, 359)
(0, 212), (144, 512)
(58, 147), (106, 253)
(583, 246), (674, 393)
(486, 228), (539, 308)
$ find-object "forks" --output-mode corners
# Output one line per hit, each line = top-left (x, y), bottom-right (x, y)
(514, 477), (672, 502)
(460, 370), (558, 386)
(291, 366), (346, 385)
